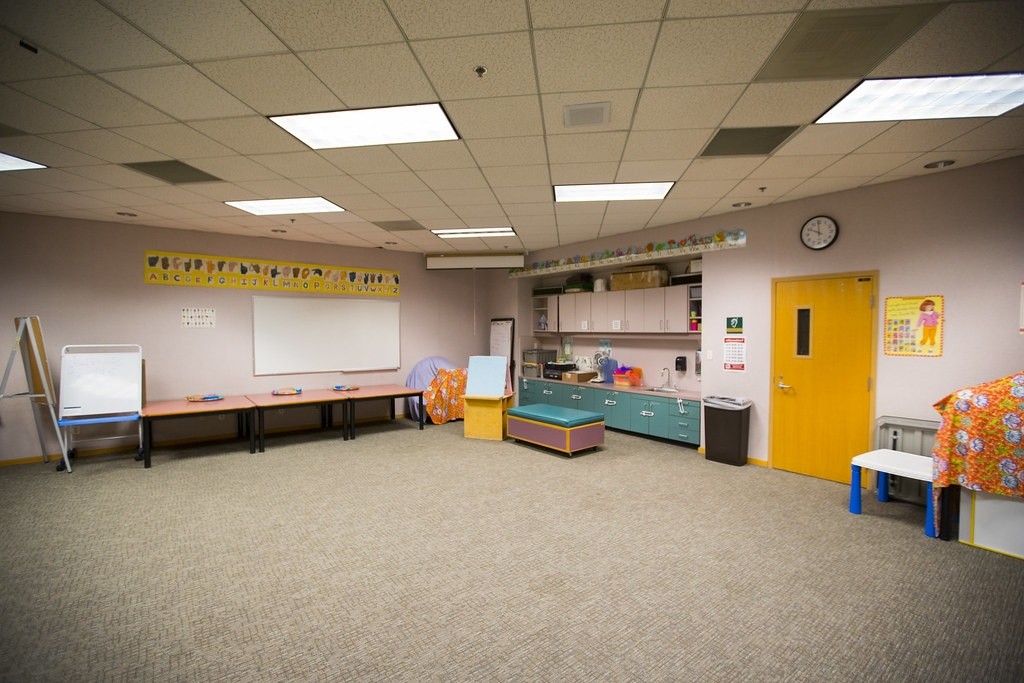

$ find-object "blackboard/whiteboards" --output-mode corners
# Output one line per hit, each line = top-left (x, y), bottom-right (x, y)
(59, 344), (142, 416)
(251, 294), (402, 376)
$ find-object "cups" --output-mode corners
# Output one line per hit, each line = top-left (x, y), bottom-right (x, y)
(691, 311), (697, 317)
(692, 320), (698, 331)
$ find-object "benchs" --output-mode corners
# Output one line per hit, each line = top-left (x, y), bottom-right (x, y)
(851, 448), (935, 537)
(507, 403), (605, 457)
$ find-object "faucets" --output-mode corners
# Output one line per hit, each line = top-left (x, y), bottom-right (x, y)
(661, 367), (670, 386)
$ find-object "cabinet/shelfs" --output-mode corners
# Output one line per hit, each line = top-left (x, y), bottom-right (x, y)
(669, 398), (701, 445)
(575, 291), (607, 333)
(594, 388), (630, 431)
(688, 283), (702, 333)
(630, 393), (669, 439)
(561, 384), (593, 413)
(519, 378), (534, 406)
(958, 486), (1024, 560)
(547, 293), (575, 333)
(534, 381), (561, 406)
(530, 296), (547, 332)
(461, 392), (516, 441)
(607, 288), (644, 333)
(645, 284), (688, 333)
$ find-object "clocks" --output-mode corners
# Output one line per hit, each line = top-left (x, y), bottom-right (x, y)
(800, 215), (840, 251)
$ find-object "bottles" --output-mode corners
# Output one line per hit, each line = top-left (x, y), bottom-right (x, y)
(539, 314), (547, 331)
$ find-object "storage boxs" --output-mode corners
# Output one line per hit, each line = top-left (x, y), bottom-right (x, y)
(612, 374), (640, 387)
(523, 349), (557, 377)
(611, 264), (669, 291)
(561, 371), (598, 383)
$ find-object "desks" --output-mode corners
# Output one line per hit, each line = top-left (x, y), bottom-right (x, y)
(141, 383), (424, 468)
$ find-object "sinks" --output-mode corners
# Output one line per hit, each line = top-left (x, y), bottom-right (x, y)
(642, 388), (678, 393)
